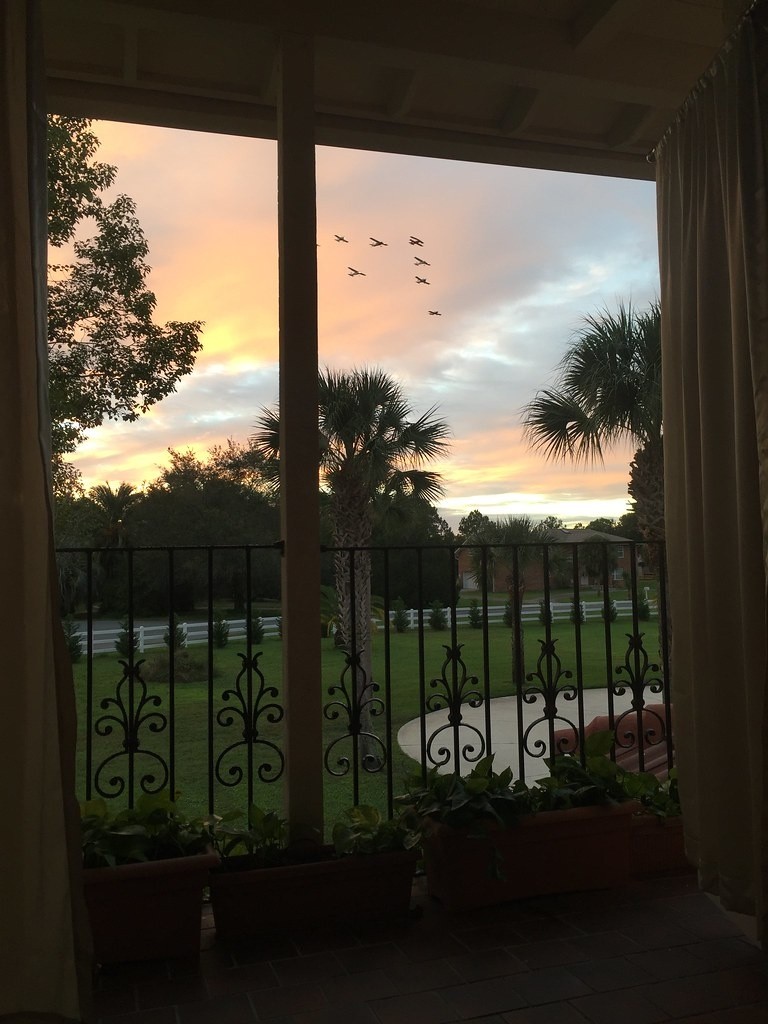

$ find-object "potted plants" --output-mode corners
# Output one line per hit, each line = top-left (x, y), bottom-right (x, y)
(628, 768), (692, 880)
(78, 788), (222, 963)
(207, 803), (423, 945)
(393, 728), (664, 917)
